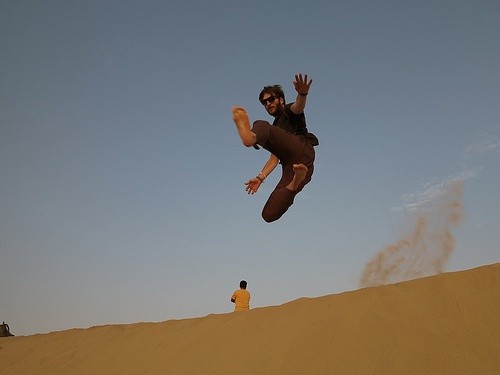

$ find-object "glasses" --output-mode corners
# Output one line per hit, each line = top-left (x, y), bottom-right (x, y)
(261, 96), (279, 105)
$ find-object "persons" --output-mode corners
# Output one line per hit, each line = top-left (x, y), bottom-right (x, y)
(232, 73), (319, 223)
(231, 280), (251, 312)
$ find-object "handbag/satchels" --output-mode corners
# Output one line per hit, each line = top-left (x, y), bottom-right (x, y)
(0, 321), (14, 337)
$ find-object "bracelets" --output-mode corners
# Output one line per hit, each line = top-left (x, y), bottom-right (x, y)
(259, 172), (266, 178)
(298, 93), (308, 96)
(256, 177), (264, 183)
